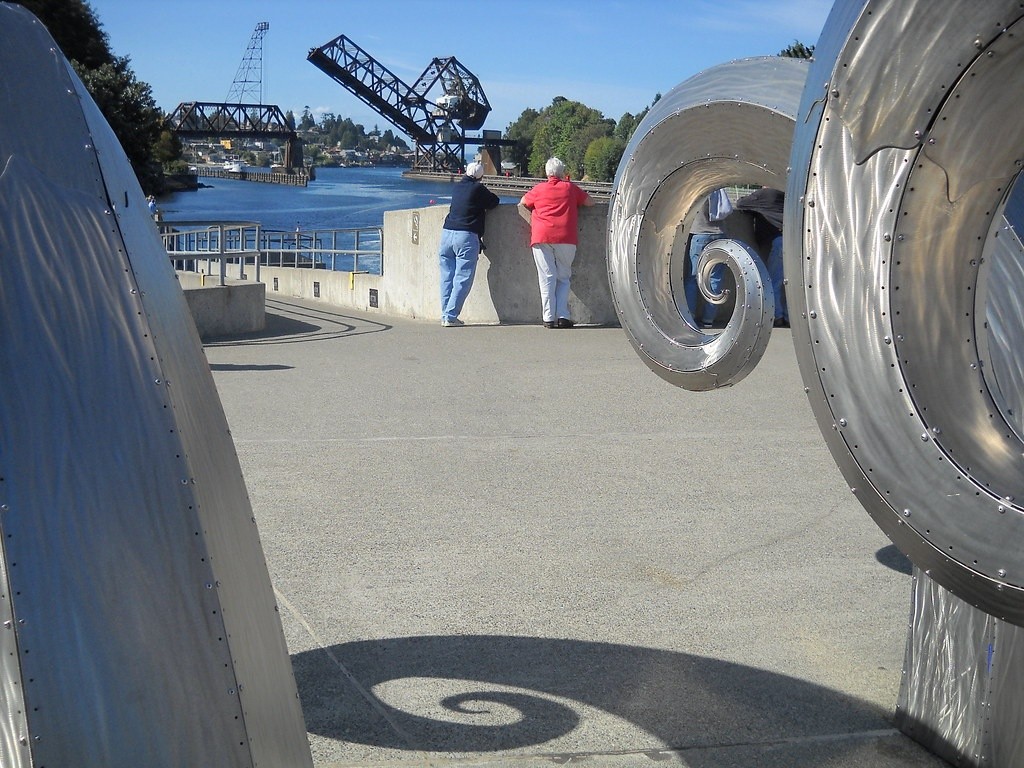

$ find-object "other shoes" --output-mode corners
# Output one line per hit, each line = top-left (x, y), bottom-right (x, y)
(557, 317), (573, 328)
(440, 319), (445, 327)
(445, 318), (465, 326)
(542, 321), (554, 329)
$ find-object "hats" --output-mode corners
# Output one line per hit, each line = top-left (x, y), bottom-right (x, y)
(466, 161), (484, 179)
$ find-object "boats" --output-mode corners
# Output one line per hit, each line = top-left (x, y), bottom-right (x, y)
(188, 163), (198, 173)
(222, 160), (243, 174)
(269, 163), (283, 169)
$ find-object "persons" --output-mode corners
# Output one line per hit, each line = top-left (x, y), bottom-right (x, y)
(520, 157), (595, 329)
(146, 195), (156, 217)
(439, 162), (500, 326)
(685, 187), (790, 327)
